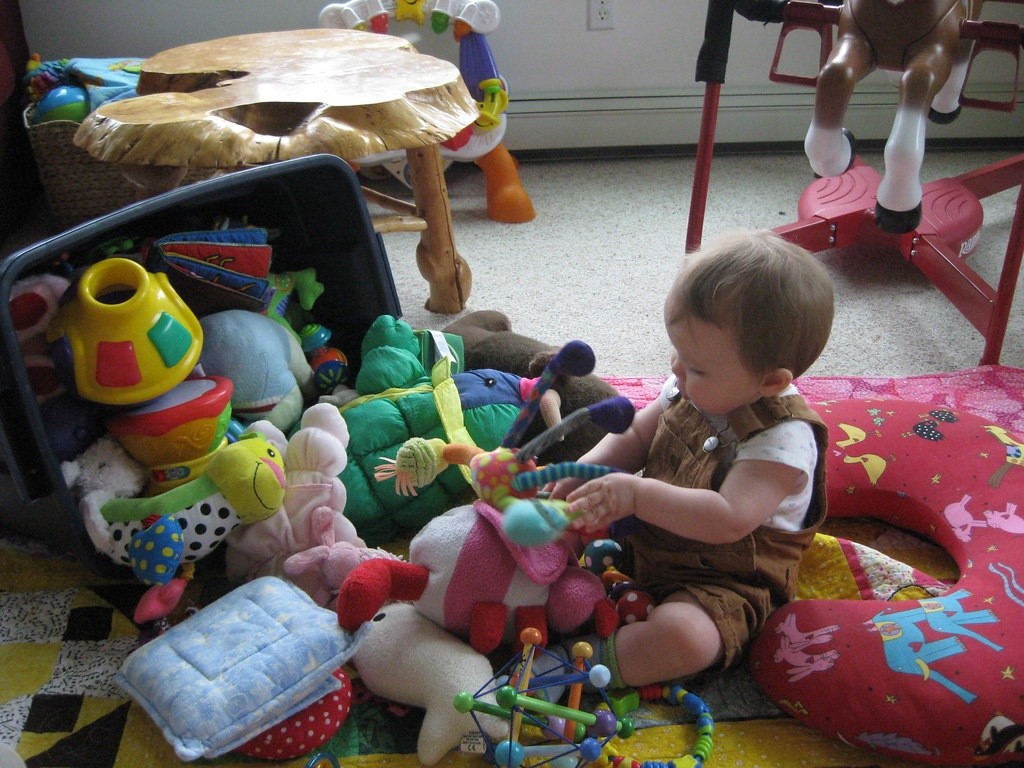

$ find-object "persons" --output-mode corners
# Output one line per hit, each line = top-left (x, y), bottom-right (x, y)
(511, 232), (835, 705)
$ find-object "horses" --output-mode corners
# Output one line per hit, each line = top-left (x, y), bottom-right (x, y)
(802, 0), (984, 236)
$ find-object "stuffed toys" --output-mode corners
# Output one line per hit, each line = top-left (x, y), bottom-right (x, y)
(7, 227), (635, 768)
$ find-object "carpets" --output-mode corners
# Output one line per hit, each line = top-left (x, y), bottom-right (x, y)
(318, 363), (1024, 768)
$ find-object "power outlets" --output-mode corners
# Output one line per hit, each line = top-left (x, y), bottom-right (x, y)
(587, 0), (614, 29)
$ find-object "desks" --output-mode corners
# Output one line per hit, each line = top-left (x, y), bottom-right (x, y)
(73, 27), (479, 315)
